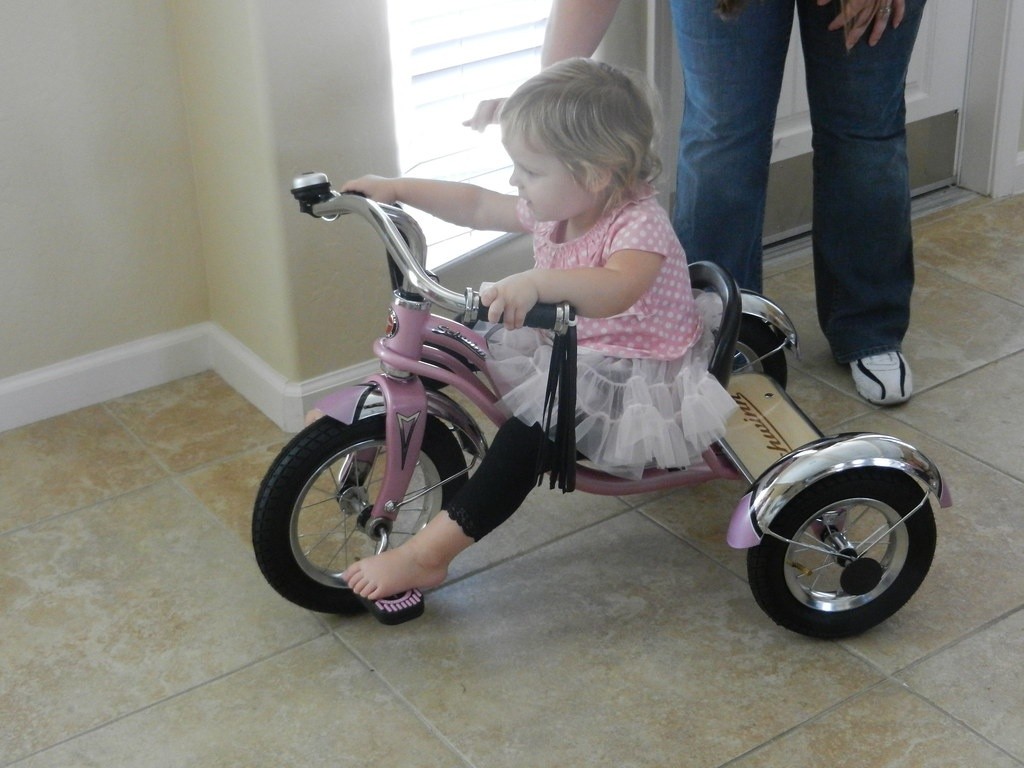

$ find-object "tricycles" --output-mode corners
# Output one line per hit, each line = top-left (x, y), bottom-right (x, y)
(249, 169), (954, 641)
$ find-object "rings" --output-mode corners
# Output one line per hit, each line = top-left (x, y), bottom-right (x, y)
(879, 7), (891, 13)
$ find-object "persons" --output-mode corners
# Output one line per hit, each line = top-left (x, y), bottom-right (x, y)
(339, 57), (740, 601)
(462, 0), (929, 406)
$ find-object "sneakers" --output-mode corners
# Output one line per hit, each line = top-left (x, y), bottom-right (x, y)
(849, 351), (912, 404)
